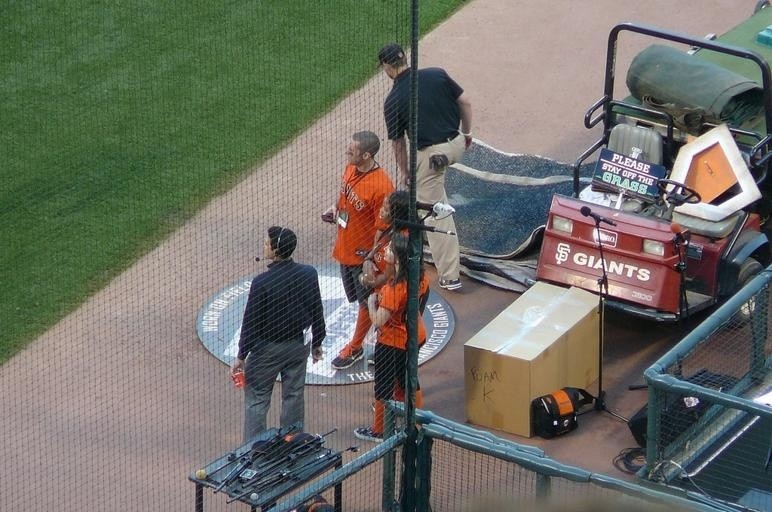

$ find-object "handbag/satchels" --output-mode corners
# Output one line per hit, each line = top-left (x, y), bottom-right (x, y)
(533, 386), (580, 439)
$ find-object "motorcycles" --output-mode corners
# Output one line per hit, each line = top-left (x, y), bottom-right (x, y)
(533, 22), (771, 330)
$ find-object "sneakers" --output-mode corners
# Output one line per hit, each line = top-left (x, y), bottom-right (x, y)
(354, 427), (384, 442)
(331, 344), (364, 369)
(438, 277), (462, 290)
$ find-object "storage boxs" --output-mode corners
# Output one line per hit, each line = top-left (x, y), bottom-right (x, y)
(463, 279), (605, 439)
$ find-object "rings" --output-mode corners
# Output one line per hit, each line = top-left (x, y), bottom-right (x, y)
(364, 274), (367, 277)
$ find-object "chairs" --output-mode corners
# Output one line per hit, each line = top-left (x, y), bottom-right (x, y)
(579, 123), (746, 238)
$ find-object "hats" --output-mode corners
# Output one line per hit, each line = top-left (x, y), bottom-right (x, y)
(379, 44), (402, 67)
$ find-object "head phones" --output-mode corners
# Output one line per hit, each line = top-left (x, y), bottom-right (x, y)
(273, 226), (287, 256)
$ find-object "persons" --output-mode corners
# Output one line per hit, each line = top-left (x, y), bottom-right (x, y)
(227, 226), (328, 446)
(352, 231), (424, 444)
(355, 189), (430, 408)
(372, 42), (474, 292)
(322, 131), (395, 371)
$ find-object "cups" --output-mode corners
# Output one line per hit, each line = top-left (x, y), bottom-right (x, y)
(231, 371), (247, 389)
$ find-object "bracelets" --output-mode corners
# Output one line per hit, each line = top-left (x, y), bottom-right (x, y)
(362, 257), (372, 263)
(360, 276), (363, 286)
(460, 130), (472, 136)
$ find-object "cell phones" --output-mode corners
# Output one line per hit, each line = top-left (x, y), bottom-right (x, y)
(321, 214), (334, 222)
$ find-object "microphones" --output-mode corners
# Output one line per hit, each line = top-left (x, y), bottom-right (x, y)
(670, 222), (689, 248)
(580, 206), (617, 226)
(255, 257), (275, 262)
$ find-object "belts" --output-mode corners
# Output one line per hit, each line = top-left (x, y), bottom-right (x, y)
(436, 131), (459, 144)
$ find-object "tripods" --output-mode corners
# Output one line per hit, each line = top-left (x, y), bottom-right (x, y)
(575, 223), (629, 422)
(629, 248), (708, 389)
